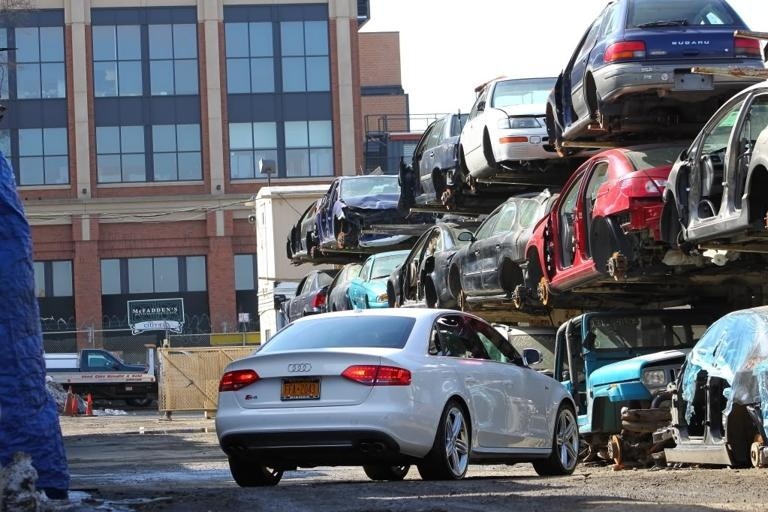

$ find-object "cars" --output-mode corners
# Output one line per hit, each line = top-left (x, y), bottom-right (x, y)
(214, 307), (579, 487)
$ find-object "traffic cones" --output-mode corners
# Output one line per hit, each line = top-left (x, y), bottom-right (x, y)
(62, 385), (95, 417)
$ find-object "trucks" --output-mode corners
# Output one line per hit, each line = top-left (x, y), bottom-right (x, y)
(546, 312), (697, 436)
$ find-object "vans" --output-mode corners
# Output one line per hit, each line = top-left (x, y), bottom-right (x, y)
(42, 354), (115, 369)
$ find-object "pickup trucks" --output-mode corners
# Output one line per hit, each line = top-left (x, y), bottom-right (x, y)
(43, 350), (154, 408)
